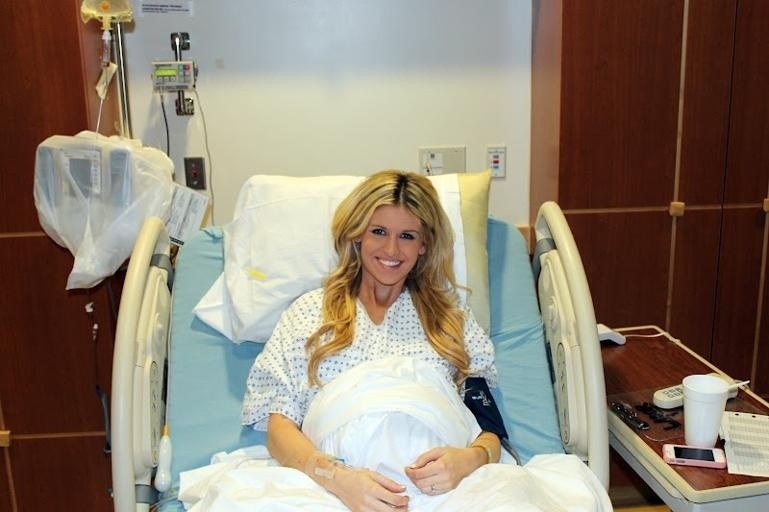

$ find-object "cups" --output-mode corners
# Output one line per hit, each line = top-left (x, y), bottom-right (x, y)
(682, 374), (727, 448)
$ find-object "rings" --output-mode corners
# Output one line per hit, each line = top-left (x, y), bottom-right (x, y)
(431, 484), (436, 492)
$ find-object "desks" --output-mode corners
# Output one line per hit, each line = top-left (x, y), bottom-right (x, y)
(597, 322), (769, 510)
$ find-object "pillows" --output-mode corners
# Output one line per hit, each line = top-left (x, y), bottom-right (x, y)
(230, 172), (496, 342)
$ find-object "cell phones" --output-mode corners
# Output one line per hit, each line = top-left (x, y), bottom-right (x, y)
(662, 443), (727, 469)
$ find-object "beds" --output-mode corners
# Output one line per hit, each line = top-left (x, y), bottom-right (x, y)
(106, 202), (611, 512)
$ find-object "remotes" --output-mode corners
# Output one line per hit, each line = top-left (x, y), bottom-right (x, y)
(653, 372), (738, 409)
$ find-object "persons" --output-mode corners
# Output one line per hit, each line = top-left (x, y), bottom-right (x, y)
(239, 169), (510, 512)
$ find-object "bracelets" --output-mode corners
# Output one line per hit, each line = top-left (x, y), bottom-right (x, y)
(471, 443), (493, 464)
